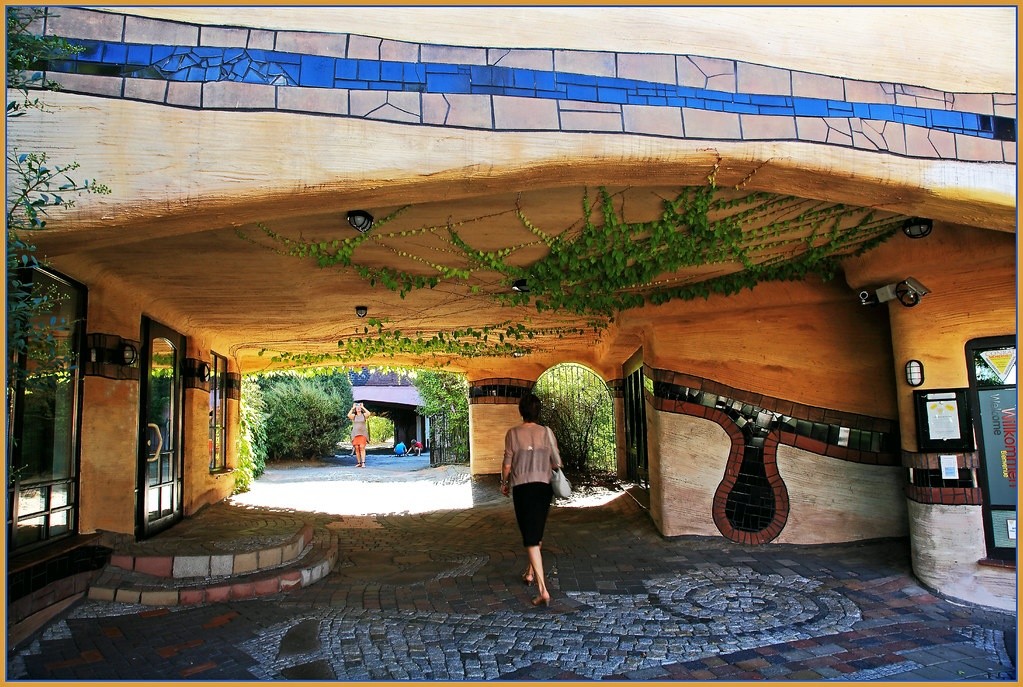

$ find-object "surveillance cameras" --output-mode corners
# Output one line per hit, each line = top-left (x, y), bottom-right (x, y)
(905, 276), (931, 298)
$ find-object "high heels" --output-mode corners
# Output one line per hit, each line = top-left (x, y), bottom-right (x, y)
(521, 570), (534, 587)
(531, 594), (551, 607)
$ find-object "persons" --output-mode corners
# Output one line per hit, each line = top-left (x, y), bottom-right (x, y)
(501, 394), (563, 607)
(347, 402), (371, 468)
(405, 439), (424, 456)
(393, 440), (409, 457)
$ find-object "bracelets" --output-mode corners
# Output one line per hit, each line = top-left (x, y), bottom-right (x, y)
(500, 480), (509, 486)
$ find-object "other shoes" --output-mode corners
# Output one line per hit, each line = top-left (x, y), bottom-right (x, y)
(355, 462), (366, 468)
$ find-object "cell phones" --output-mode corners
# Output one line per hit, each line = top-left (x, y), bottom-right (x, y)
(355, 403), (364, 408)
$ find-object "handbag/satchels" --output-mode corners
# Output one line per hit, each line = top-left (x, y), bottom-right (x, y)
(544, 426), (572, 500)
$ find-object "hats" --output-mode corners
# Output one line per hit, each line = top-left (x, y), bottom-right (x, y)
(411, 439), (416, 444)
